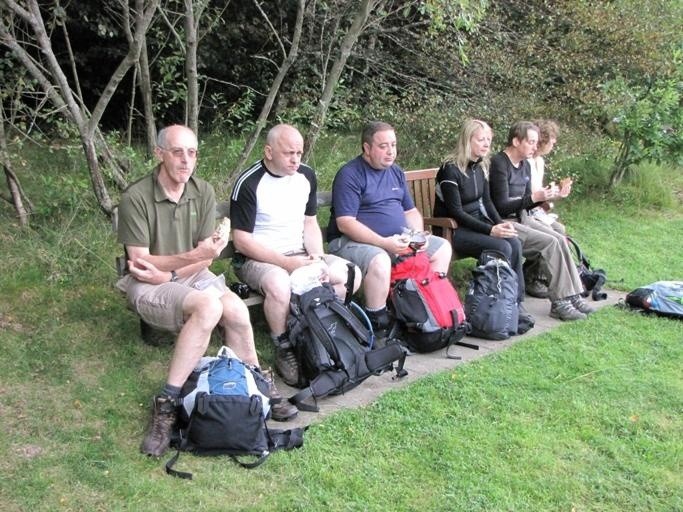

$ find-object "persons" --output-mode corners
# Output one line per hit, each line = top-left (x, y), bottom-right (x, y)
(525, 120), (602, 298)
(430, 118), (533, 325)
(322, 120), (453, 341)
(229, 124), (364, 387)
(489, 121), (593, 322)
(117, 123), (297, 458)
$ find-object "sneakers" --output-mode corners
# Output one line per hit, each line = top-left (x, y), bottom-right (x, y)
(259, 368), (299, 421)
(275, 340), (308, 387)
(140, 396), (179, 455)
(551, 294), (596, 320)
(526, 280), (550, 297)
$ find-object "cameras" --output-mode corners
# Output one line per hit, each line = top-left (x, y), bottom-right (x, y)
(230, 281), (250, 299)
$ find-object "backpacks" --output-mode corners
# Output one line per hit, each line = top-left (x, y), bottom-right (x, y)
(625, 280), (683, 317)
(388, 247), (468, 352)
(564, 236), (605, 294)
(467, 249), (520, 339)
(182, 344), (268, 456)
(288, 281), (407, 412)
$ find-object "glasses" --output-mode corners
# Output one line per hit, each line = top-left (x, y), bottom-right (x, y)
(158, 145), (197, 158)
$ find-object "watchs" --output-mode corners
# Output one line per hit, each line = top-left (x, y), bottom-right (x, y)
(169, 270), (179, 283)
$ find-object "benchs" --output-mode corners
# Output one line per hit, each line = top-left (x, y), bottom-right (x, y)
(113, 189), (338, 348)
(401, 167), (553, 275)
